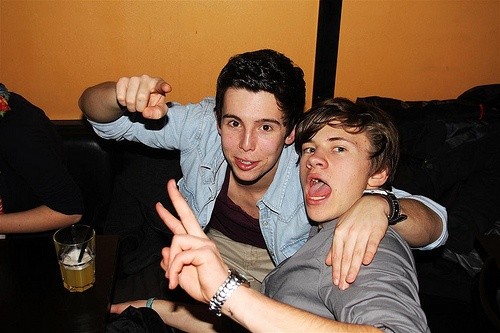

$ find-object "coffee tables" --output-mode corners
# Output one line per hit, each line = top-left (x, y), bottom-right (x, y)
(0, 232), (123, 333)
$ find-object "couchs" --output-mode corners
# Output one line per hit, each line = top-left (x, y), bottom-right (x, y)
(48, 83), (500, 333)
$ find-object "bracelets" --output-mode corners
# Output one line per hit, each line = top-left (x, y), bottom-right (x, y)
(146, 297), (158, 308)
(363, 188), (409, 225)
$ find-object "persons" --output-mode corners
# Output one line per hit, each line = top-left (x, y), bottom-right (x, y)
(77, 48), (448, 289)
(0, 82), (83, 233)
(109, 96), (431, 333)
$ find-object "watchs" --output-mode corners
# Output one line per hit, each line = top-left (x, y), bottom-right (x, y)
(209, 268), (251, 316)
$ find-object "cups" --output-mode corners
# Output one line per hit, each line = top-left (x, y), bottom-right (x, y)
(52, 224), (96, 292)
